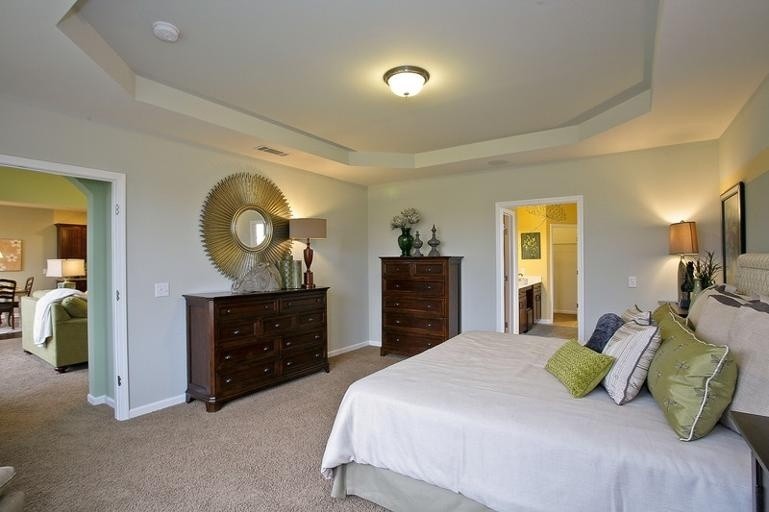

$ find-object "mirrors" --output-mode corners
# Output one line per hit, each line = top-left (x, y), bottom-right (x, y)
(199, 172), (294, 281)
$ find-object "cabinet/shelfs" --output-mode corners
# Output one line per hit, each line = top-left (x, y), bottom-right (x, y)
(54, 223), (87, 292)
(519, 282), (541, 334)
(378, 256), (464, 356)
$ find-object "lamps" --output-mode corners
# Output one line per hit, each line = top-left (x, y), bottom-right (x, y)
(669, 220), (699, 305)
(45, 259), (85, 289)
(383, 65), (430, 97)
(289, 218), (327, 288)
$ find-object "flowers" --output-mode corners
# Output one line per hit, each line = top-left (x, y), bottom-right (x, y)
(391, 208), (420, 230)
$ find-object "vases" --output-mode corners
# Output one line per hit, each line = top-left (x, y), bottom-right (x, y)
(397, 228), (414, 257)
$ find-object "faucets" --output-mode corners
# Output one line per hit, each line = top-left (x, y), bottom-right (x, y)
(518, 273), (523, 276)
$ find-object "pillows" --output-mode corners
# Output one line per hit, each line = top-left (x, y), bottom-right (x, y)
(32, 289), (87, 318)
(646, 300), (739, 444)
(585, 301), (661, 407)
(545, 338), (616, 399)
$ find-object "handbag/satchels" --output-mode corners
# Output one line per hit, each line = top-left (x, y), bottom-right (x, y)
(231, 261), (282, 293)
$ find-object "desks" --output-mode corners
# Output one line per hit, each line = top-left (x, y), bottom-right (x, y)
(182, 286), (329, 412)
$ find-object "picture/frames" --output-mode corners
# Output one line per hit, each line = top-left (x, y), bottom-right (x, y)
(720, 181), (746, 283)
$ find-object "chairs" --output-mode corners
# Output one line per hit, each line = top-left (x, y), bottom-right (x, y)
(0, 276), (34, 329)
(0, 466), (26, 512)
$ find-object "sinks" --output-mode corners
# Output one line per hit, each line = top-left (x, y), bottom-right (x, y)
(518, 277), (528, 287)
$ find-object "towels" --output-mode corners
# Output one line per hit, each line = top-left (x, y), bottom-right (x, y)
(33, 288), (87, 348)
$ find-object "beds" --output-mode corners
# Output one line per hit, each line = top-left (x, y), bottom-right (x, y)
(321, 252), (769, 512)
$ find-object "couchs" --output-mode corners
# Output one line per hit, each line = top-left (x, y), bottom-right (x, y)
(21, 296), (88, 373)
